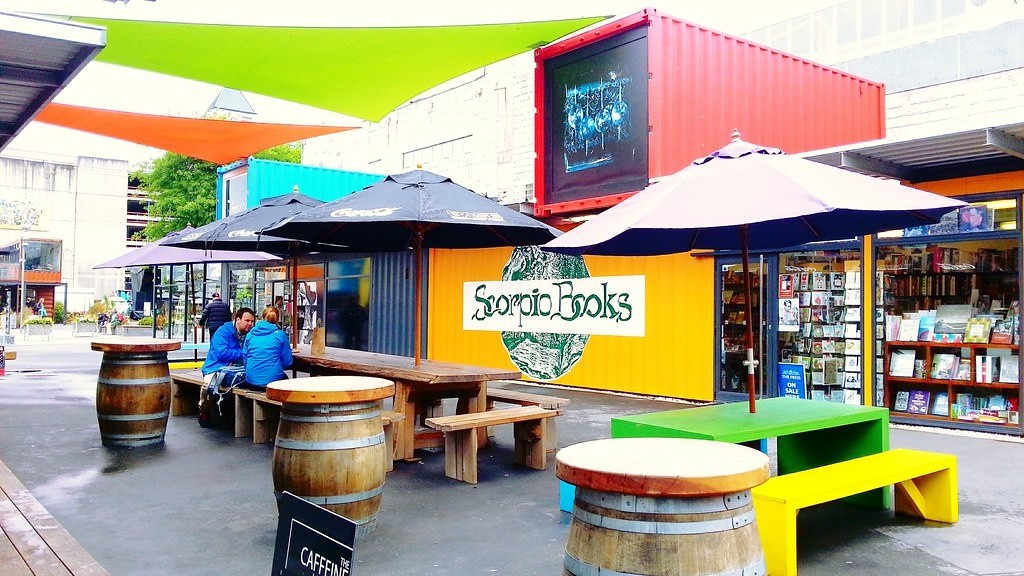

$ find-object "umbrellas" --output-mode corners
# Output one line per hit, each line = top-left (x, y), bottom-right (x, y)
(159, 184), (354, 349)
(92, 220), (285, 361)
(253, 163), (567, 367)
(537, 126), (970, 413)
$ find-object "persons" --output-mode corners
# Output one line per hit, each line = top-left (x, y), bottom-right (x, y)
(242, 306), (293, 392)
(25, 296), (45, 318)
(274, 295), (286, 331)
(198, 292), (232, 343)
(201, 307), (256, 387)
(130, 310), (142, 321)
(97, 307), (121, 334)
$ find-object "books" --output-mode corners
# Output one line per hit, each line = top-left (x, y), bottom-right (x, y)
(886, 304), (1020, 423)
(724, 268), (759, 354)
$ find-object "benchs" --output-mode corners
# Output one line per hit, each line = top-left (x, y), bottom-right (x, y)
(425, 405), (563, 485)
(170, 370), (260, 437)
(750, 448), (958, 576)
(121, 325), (153, 337)
(560, 448), (576, 525)
(486, 387), (572, 453)
(245, 393), (406, 472)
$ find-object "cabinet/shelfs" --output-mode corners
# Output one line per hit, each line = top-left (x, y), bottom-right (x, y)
(721, 245), (1019, 427)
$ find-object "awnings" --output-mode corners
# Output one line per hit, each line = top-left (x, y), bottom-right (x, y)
(19, 13), (615, 167)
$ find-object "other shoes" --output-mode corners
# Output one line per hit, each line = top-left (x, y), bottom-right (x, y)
(198, 408), (210, 429)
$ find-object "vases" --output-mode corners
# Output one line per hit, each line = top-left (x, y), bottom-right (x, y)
(73, 323), (96, 333)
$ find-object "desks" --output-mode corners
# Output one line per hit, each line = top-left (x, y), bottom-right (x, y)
(283, 344), (522, 461)
(610, 395), (890, 509)
(265, 376), (395, 526)
(91, 338), (181, 448)
(553, 436), (771, 576)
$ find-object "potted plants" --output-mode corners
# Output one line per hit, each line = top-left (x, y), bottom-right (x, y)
(23, 316), (54, 335)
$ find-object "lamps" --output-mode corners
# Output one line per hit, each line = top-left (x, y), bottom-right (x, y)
(994, 220), (1016, 230)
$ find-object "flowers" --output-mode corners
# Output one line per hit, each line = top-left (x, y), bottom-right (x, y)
(72, 316), (97, 323)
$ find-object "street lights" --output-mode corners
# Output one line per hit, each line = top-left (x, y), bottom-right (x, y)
(19, 242), (30, 330)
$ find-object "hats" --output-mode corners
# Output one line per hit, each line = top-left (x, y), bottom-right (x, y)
(212, 292), (220, 300)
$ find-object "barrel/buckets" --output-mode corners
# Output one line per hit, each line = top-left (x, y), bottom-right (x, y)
(96, 351), (171, 448)
(271, 401), (389, 539)
(560, 487), (772, 576)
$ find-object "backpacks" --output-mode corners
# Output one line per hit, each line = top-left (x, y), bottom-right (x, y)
(208, 363), (247, 416)
